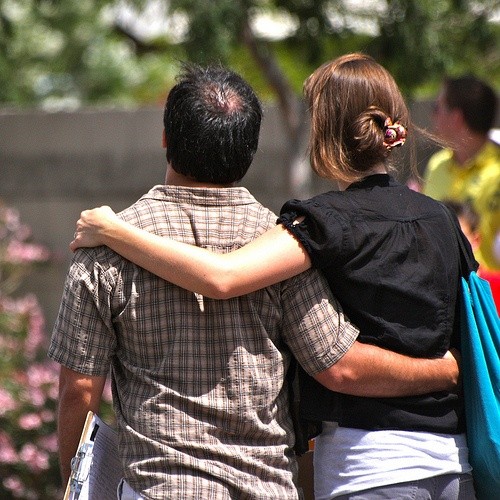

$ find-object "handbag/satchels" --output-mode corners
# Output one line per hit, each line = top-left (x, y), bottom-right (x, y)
(437, 201), (500, 500)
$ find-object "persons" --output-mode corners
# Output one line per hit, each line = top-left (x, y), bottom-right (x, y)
(423, 71), (499, 268)
(45, 60), (465, 500)
(67, 52), (477, 500)
